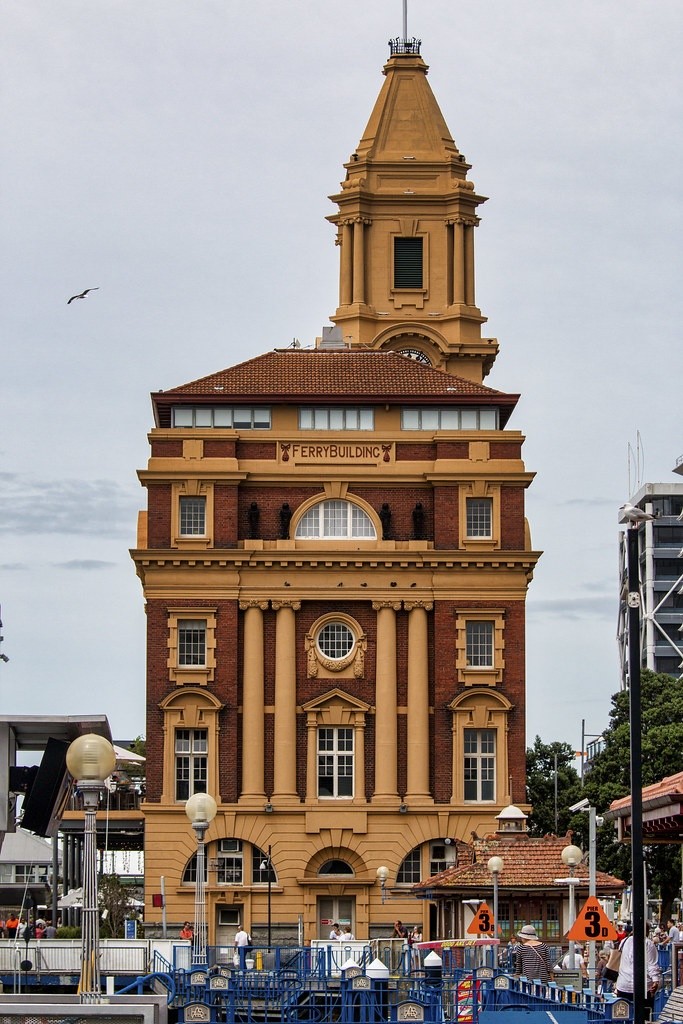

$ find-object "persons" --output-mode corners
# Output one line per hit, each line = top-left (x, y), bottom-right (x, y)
(179, 921), (193, 941)
(625, 920), (632, 937)
(234, 925), (252, 969)
(616, 921), (660, 1024)
(330, 923), (355, 941)
(43, 921), (57, 939)
(597, 950), (608, 978)
(659, 922), (669, 943)
(659, 919), (679, 945)
(561, 949), (586, 975)
(18, 918), (27, 939)
(6, 914), (19, 939)
(602, 942), (621, 983)
(508, 937), (520, 952)
(652, 927), (663, 946)
(57, 917), (62, 928)
(36, 923), (46, 939)
(391, 920), (423, 944)
(36, 915), (46, 926)
(516, 925), (554, 984)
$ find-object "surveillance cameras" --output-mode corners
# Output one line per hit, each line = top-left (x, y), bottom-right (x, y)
(260, 863), (265, 871)
(597, 817), (603, 826)
(569, 799), (589, 812)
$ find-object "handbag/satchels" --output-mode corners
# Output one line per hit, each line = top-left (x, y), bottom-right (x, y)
(408, 931), (415, 945)
(234, 952), (240, 969)
(247, 933), (253, 953)
(602, 935), (631, 981)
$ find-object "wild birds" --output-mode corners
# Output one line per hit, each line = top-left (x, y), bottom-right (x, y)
(66, 287), (99, 304)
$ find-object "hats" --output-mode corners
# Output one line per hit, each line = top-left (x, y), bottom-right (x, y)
(517, 925), (539, 941)
(655, 928), (662, 935)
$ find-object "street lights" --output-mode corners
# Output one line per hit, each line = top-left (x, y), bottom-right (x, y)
(66, 734), (116, 995)
(488, 856), (505, 969)
(185, 793), (218, 963)
(561, 845), (584, 968)
(259, 844), (272, 953)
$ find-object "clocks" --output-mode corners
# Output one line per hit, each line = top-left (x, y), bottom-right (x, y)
(396, 349), (432, 367)
